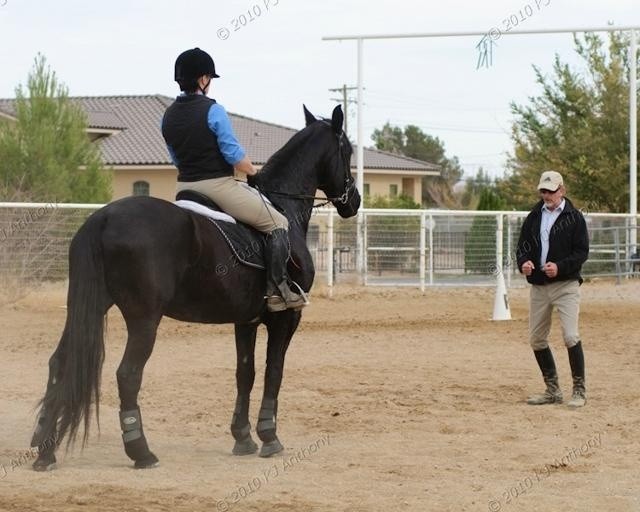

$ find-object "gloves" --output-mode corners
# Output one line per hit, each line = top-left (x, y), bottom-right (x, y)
(247, 172), (256, 186)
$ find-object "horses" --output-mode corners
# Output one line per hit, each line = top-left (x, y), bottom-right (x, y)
(31, 102), (362, 472)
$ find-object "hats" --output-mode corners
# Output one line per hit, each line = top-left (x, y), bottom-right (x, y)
(174, 48), (219, 80)
(537, 170), (563, 192)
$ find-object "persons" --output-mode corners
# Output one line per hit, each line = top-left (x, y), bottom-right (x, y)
(160, 48), (311, 312)
(516, 170), (589, 407)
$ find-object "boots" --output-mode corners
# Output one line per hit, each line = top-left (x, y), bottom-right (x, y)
(567, 342), (586, 409)
(265, 229), (305, 312)
(526, 348), (563, 404)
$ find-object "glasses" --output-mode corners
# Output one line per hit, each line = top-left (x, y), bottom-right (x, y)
(539, 188), (560, 193)
